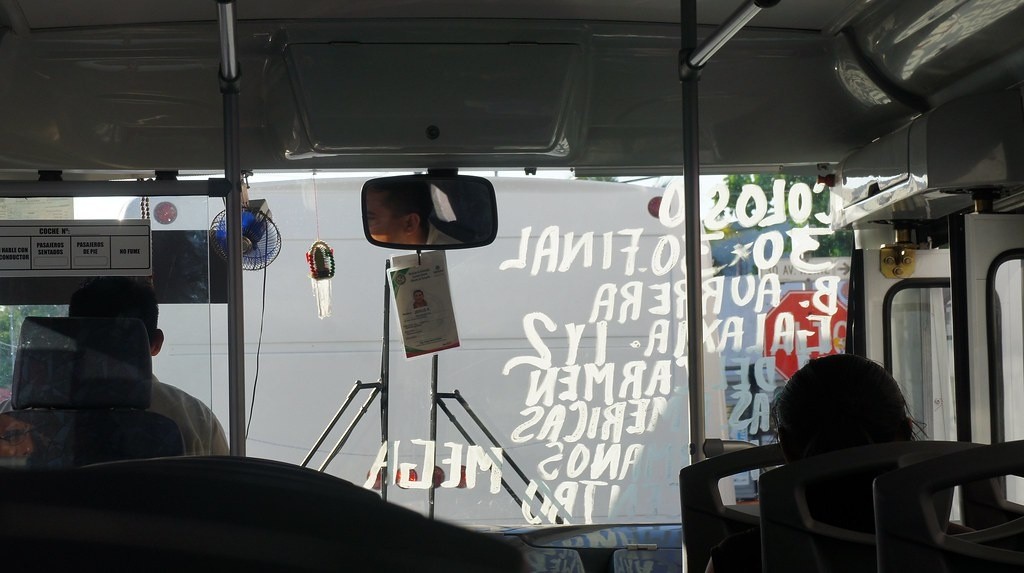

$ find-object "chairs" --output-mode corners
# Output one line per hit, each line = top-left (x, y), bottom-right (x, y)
(0, 314), (186, 467)
(679, 436), (1024, 573)
(0, 455), (529, 572)
(422, 176), (491, 241)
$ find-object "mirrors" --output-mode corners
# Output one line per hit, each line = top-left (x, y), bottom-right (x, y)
(362, 173), (497, 250)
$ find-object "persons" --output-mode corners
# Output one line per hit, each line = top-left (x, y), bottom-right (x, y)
(0, 408), (63, 464)
(364, 176), (465, 246)
(703, 353), (976, 573)
(413, 289), (427, 309)
(66, 267), (231, 459)
